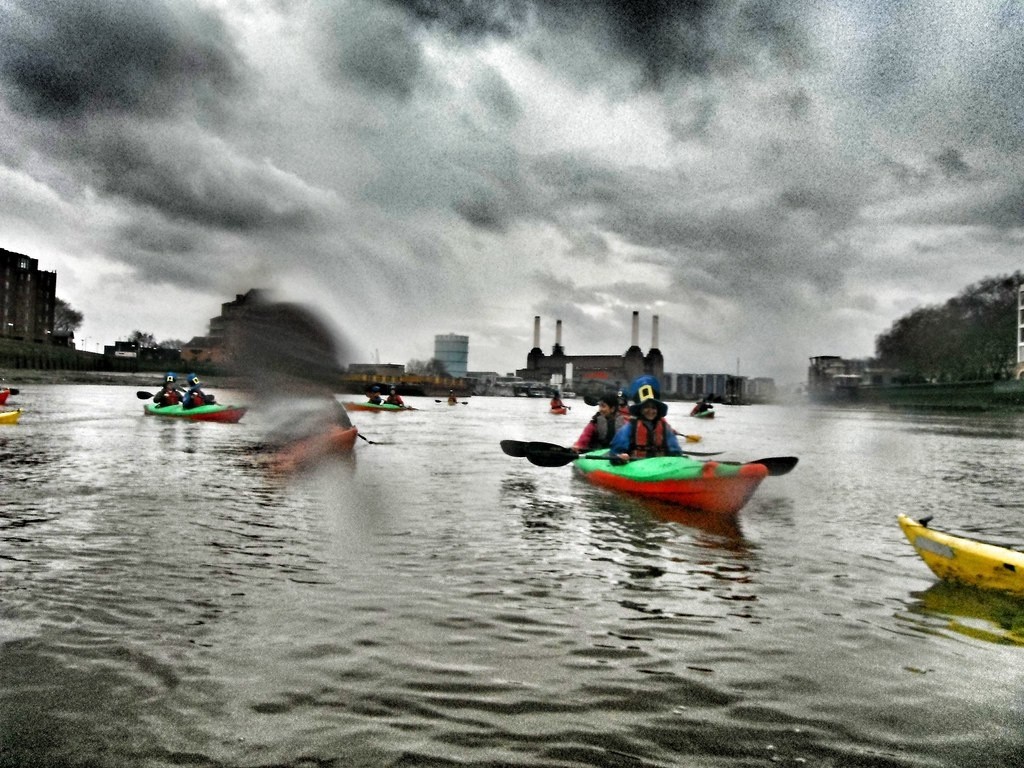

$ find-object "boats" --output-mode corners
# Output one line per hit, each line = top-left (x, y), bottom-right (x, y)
(0, 386), (20, 404)
(260, 424), (358, 473)
(144, 401), (251, 424)
(447, 399), (457, 406)
(550, 408), (567, 415)
(342, 400), (418, 412)
(693, 410), (715, 419)
(570, 448), (769, 515)
(895, 511), (1024, 599)
(0, 407), (22, 425)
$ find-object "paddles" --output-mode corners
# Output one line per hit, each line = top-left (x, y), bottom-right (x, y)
(695, 393), (714, 415)
(674, 432), (702, 443)
(136, 390), (154, 400)
(356, 433), (375, 445)
(178, 386), (223, 406)
(526, 440), (802, 476)
(583, 394), (632, 415)
(562, 405), (571, 410)
(499, 438), (725, 459)
(434, 399), (469, 405)
(365, 391), (388, 402)
(9, 388), (19, 395)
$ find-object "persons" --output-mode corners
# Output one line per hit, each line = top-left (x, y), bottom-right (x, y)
(694, 399), (713, 415)
(550, 392), (562, 408)
(573, 394), (624, 451)
(365, 386), (381, 404)
(387, 390), (403, 404)
(183, 373), (216, 409)
(611, 375), (682, 462)
(154, 372), (183, 406)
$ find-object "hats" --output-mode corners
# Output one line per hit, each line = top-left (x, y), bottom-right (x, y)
(627, 375), (668, 418)
(186, 373), (203, 391)
(161, 372), (180, 391)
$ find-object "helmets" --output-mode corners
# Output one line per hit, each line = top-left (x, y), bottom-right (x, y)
(389, 386), (397, 395)
(371, 385), (382, 393)
